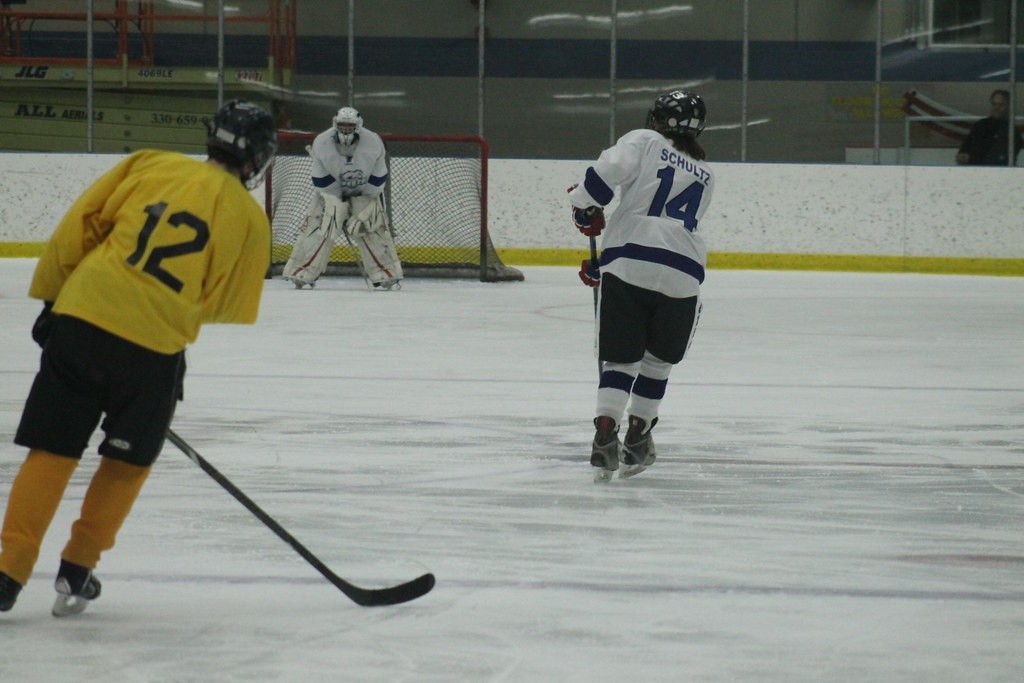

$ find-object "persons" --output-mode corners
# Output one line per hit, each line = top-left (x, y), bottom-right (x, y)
(567, 90), (715, 485)
(1, 98), (280, 619)
(279, 106), (406, 291)
(955, 89), (1024, 167)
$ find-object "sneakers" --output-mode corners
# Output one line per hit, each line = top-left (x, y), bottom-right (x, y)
(49, 560), (102, 617)
(0, 573), (23, 611)
(590, 413), (657, 482)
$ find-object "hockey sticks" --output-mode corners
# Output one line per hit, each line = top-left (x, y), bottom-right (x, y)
(584, 204), (601, 364)
(340, 222), (404, 288)
(163, 426), (437, 608)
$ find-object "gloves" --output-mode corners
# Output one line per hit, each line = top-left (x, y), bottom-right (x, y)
(579, 259), (601, 286)
(30, 301), (54, 348)
(571, 204), (606, 236)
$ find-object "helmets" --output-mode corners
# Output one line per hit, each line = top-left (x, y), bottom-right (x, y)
(332, 107), (363, 146)
(201, 101), (278, 189)
(645, 90), (708, 137)
